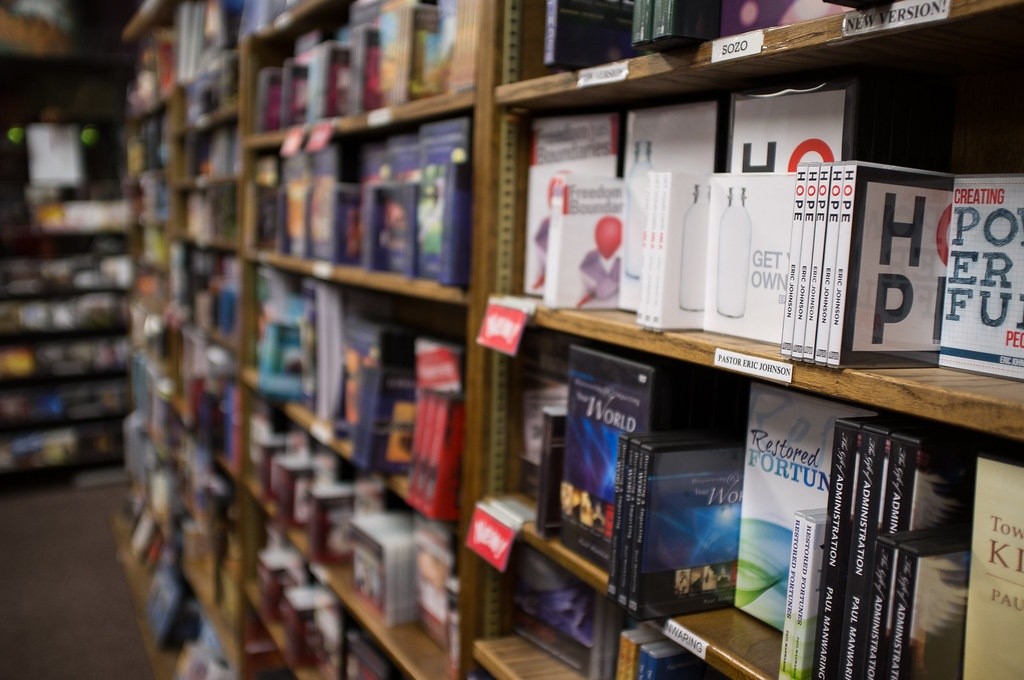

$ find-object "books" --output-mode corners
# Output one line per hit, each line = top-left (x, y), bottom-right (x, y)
(3, 1), (1023, 680)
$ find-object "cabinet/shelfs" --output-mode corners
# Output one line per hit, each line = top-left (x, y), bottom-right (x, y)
(0, 0), (1024, 678)
